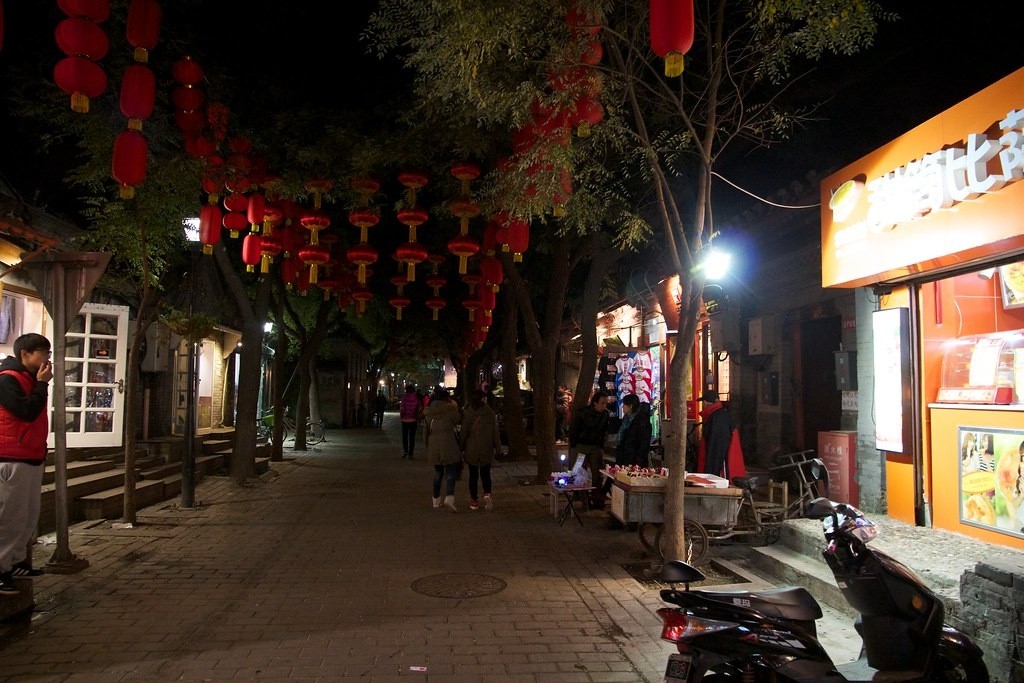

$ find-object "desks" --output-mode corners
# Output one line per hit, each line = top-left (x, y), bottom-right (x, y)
(547, 480), (597, 528)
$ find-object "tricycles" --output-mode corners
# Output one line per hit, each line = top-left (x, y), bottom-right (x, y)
(598, 450), (823, 566)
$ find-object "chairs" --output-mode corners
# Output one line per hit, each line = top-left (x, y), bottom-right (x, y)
(745, 479), (788, 532)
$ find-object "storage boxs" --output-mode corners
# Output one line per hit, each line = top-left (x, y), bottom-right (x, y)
(617, 471), (669, 487)
(687, 472), (730, 489)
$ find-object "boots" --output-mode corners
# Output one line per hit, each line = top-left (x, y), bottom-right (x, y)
(432, 496), (442, 508)
(444, 495), (457, 513)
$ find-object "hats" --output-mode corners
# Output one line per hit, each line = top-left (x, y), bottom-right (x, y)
(697, 390), (719, 403)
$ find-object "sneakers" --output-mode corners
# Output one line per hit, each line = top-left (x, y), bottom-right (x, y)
(484, 494), (493, 512)
(0, 579), (20, 594)
(469, 500), (478, 510)
(5, 563), (45, 579)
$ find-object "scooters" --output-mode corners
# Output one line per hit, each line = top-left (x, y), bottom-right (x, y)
(656, 498), (991, 683)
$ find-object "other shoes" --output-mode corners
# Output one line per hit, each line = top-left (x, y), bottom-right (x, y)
(595, 501), (605, 509)
(400, 451), (407, 458)
(409, 456), (414, 459)
(626, 523), (637, 531)
(589, 503), (593, 509)
(607, 516), (622, 528)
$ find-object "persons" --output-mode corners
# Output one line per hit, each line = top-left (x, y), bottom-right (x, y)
(424, 391), (461, 511)
(374, 391), (388, 426)
(0, 333), (53, 594)
(696, 390), (749, 544)
(399, 385), (421, 460)
(1012, 440), (1024, 535)
(555, 384), (573, 445)
(415, 386), (506, 432)
(568, 391), (609, 510)
(459, 390), (504, 512)
(962, 433), (979, 480)
(977, 434), (994, 499)
(610, 393), (652, 531)
(358, 399), (370, 425)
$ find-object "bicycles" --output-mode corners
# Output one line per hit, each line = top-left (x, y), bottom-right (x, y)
(255, 405), (325, 445)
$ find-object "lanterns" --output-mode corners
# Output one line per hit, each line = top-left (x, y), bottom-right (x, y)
(650, 0), (694, 77)
(172, 0), (607, 374)
(111, 0), (162, 200)
(53, 0), (111, 112)
(0, 0), (4, 49)
